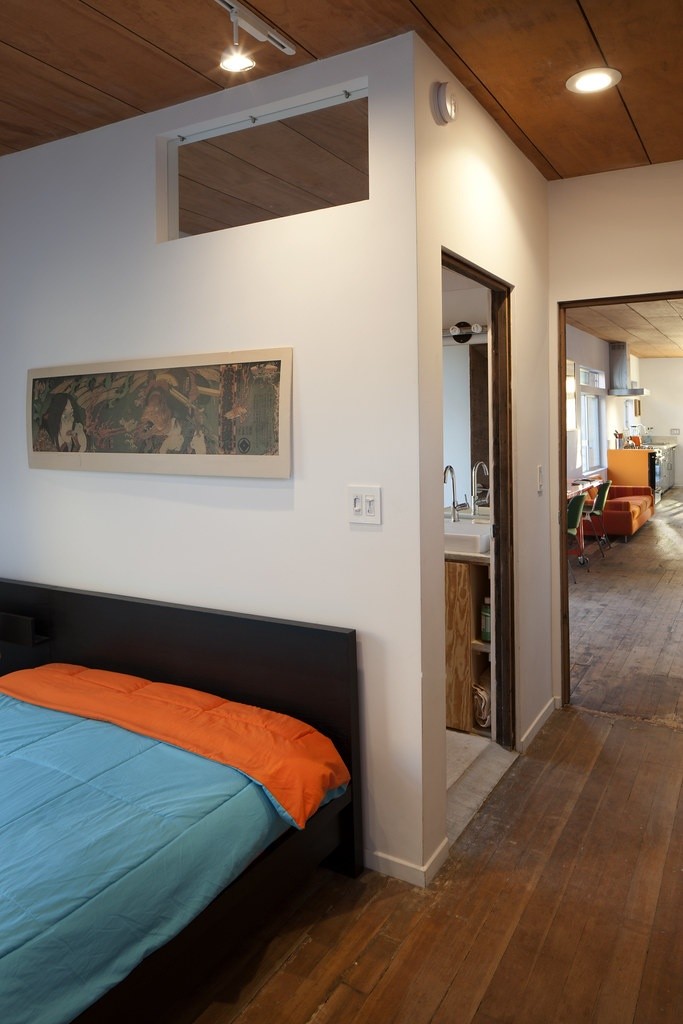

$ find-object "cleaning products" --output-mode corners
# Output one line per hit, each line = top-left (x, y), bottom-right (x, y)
(645, 426), (654, 445)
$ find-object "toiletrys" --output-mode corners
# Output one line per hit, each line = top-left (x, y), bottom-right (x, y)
(481, 597), (492, 642)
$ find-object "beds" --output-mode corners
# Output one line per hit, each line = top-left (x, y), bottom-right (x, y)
(0, 574), (367, 1023)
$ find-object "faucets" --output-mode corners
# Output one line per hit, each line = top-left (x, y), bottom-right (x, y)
(638, 424), (647, 442)
(444, 465), (470, 522)
(471, 459), (490, 515)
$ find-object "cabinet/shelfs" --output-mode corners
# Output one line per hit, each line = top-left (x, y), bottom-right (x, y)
(662, 448), (675, 492)
(443, 559), (490, 737)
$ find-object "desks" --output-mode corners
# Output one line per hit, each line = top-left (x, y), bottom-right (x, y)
(567, 477), (602, 552)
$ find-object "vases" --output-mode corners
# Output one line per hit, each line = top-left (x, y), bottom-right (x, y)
(25, 347), (294, 479)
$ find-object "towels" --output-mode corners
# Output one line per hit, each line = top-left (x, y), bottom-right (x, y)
(472, 667), (491, 728)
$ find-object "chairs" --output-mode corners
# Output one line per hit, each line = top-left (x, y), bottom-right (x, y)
(566, 494), (592, 583)
(579, 471), (656, 543)
(580, 479), (613, 558)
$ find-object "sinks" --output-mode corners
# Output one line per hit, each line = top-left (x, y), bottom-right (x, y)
(642, 442), (669, 446)
(444, 518), (491, 553)
